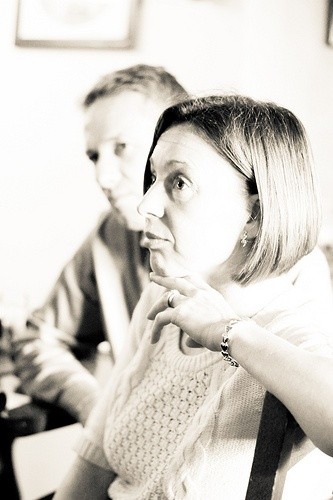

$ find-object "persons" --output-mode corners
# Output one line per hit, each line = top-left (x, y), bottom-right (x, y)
(0, 65), (190, 499)
(38, 95), (332, 500)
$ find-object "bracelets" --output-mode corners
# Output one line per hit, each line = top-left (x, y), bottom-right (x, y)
(220, 315), (255, 367)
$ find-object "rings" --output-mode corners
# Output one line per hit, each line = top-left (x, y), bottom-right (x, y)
(167, 290), (180, 307)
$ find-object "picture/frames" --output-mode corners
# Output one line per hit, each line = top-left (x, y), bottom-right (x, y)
(15, 0), (144, 48)
